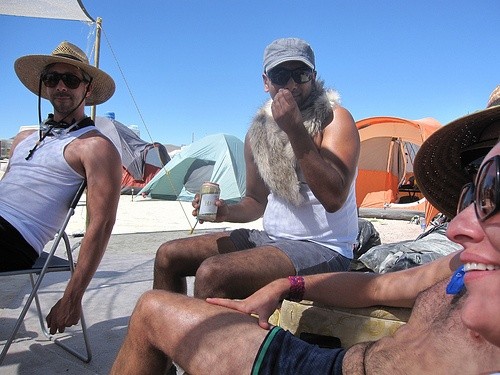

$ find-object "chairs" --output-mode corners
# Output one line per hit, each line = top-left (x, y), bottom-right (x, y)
(0, 178), (92, 364)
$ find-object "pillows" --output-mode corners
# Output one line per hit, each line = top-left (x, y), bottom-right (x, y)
(358, 221), (464, 274)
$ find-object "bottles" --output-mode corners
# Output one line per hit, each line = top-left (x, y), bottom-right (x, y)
(420, 216), (426, 233)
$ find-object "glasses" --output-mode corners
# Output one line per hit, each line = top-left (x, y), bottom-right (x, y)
(42, 71), (87, 89)
(265, 66), (315, 85)
(457, 154), (500, 221)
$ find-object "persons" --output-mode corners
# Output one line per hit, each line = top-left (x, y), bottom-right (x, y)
(152, 37), (362, 307)
(105, 86), (500, 375)
(0, 41), (124, 336)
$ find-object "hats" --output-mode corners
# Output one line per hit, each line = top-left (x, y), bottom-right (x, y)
(14, 40), (116, 106)
(411, 92), (500, 221)
(263, 38), (315, 77)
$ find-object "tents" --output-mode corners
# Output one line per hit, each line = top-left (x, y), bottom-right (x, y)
(90, 115), (246, 203)
(353, 116), (449, 228)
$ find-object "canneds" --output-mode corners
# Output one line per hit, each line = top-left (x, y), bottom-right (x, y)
(196, 181), (220, 222)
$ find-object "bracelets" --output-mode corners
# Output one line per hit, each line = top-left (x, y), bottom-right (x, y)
(286, 274), (305, 303)
